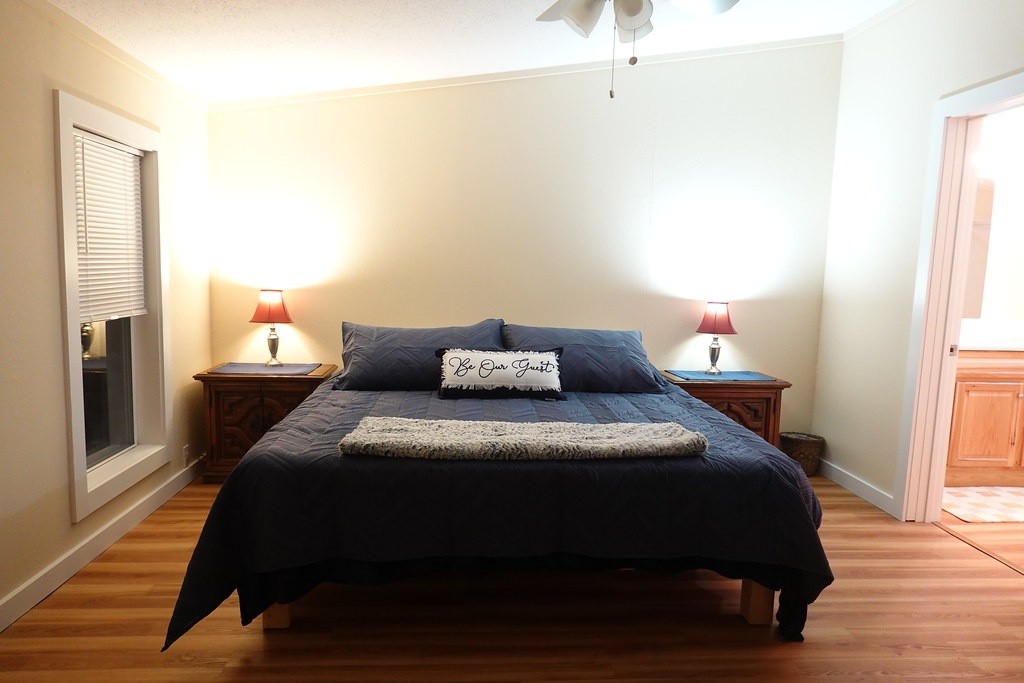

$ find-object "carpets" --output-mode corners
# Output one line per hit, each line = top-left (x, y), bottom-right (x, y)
(942, 486), (1024, 523)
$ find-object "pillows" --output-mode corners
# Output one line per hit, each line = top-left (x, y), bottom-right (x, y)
(330, 320), (506, 392)
(435, 336), (568, 401)
(501, 324), (669, 396)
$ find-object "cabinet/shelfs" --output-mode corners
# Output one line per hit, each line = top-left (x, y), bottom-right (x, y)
(944, 350), (1024, 489)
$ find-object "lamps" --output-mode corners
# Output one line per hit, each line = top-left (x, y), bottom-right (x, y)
(536, 0), (654, 98)
(249, 289), (295, 368)
(694, 300), (738, 373)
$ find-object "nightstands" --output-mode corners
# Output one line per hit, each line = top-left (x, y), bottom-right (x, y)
(81, 358), (110, 447)
(192, 362), (338, 482)
(657, 367), (792, 447)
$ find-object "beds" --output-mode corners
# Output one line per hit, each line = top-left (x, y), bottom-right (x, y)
(161, 323), (836, 654)
